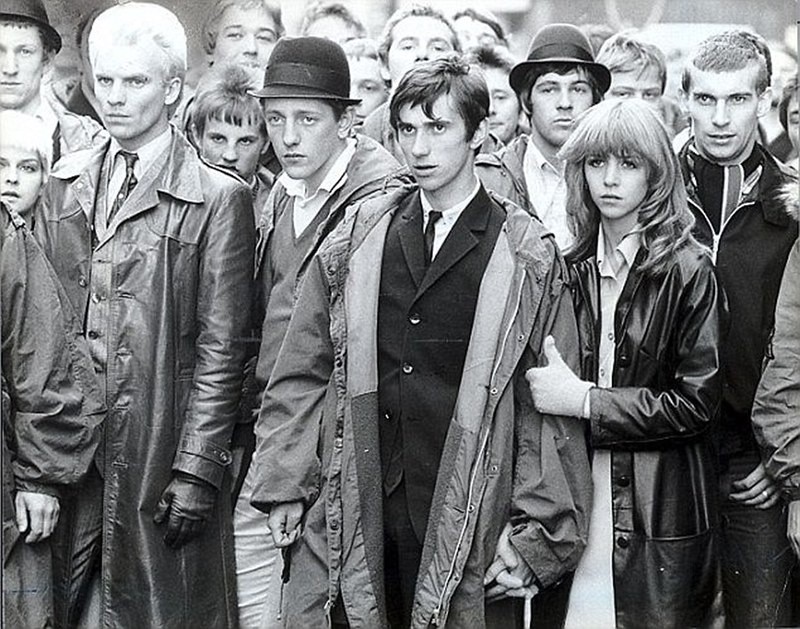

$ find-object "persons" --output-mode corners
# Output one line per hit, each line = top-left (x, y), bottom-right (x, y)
(492, 97), (732, 629)
(0, 0), (800, 629)
(31, 3), (257, 629)
(248, 52), (595, 629)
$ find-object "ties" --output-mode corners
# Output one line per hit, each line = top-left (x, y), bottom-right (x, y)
(383, 210), (443, 497)
(106, 149), (140, 229)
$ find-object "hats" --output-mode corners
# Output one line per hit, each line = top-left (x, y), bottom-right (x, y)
(509, 23), (612, 95)
(0, 0), (62, 54)
(247, 35), (363, 103)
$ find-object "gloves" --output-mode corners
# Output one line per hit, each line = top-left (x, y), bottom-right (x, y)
(494, 521), (539, 597)
(152, 471), (218, 550)
(525, 335), (597, 419)
